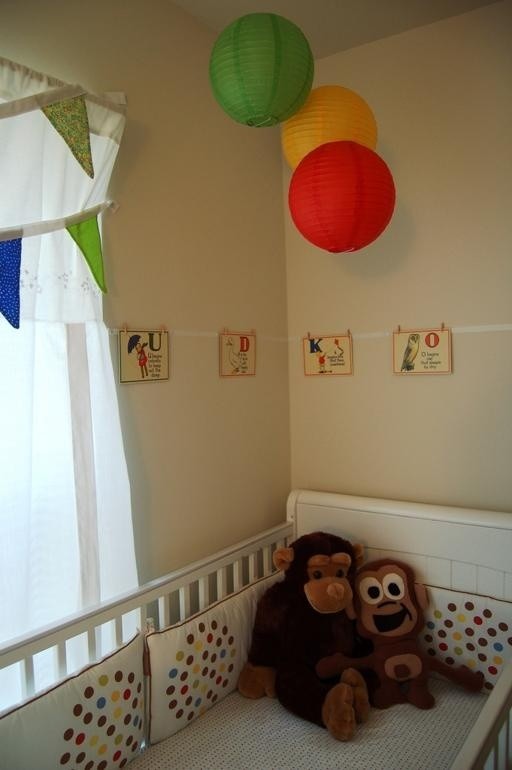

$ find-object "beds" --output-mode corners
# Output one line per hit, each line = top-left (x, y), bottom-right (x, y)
(1, 488), (512, 770)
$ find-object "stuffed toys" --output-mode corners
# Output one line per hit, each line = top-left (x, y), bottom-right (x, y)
(342, 558), (485, 708)
(238, 529), (374, 740)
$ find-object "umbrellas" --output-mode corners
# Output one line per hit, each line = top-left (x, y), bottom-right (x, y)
(126, 334), (141, 355)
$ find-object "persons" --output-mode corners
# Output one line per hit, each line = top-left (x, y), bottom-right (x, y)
(136, 343), (151, 377)
(318, 352), (326, 373)
